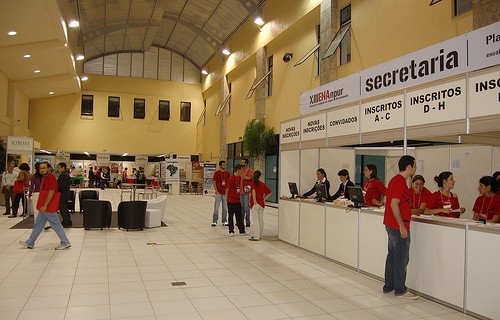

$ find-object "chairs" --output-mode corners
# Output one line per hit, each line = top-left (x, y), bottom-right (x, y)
(137, 186), (158, 200)
(117, 200), (147, 232)
(120, 185), (133, 201)
(32, 192), (51, 230)
(78, 190), (99, 213)
(144, 194), (168, 222)
(58, 190), (76, 213)
(82, 199), (112, 230)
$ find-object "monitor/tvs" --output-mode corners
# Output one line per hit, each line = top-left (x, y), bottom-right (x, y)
(288, 182), (304, 199)
(315, 183), (328, 202)
(346, 186), (366, 208)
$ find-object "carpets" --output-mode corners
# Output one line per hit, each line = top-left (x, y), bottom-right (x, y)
(9, 211), (168, 229)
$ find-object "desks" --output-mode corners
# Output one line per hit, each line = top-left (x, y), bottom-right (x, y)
(127, 178), (159, 189)
(122, 182), (147, 201)
(277, 196), (500, 320)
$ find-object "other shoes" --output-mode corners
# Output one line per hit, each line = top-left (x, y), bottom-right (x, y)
(246, 223), (250, 227)
(222, 222), (229, 225)
(240, 232), (248, 235)
(3, 212), (10, 215)
(230, 232), (234, 236)
(212, 222), (217, 226)
(249, 236), (259, 240)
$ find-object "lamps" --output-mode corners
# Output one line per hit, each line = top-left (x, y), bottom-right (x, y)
(282, 52), (293, 63)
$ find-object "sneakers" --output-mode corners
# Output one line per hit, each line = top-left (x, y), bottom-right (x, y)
(383, 290), (396, 294)
(20, 241), (34, 249)
(55, 244), (70, 250)
(396, 291), (419, 299)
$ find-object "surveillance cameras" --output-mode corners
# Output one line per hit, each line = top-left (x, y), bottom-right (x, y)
(283, 54), (291, 63)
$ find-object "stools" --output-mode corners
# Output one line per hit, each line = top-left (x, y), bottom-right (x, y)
(145, 208), (162, 228)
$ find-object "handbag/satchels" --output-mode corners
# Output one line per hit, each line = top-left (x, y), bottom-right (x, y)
(2, 186), (6, 193)
(13, 173), (27, 193)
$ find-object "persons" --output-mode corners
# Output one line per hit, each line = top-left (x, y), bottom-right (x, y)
(7, 162), (33, 218)
(381, 154), (420, 301)
(225, 164), (250, 237)
(472, 171), (500, 223)
(131, 166), (147, 189)
(329, 168), (356, 202)
(211, 160), (231, 227)
(122, 167), (129, 189)
(70, 164), (84, 176)
(359, 164), (387, 207)
(248, 170), (272, 241)
(2, 163), (21, 215)
(299, 167), (331, 202)
(56, 162), (73, 227)
(424, 170), (466, 218)
(87, 165), (110, 188)
(18, 161), (71, 250)
(28, 161), (51, 231)
(408, 174), (432, 217)
(235, 157), (256, 227)
